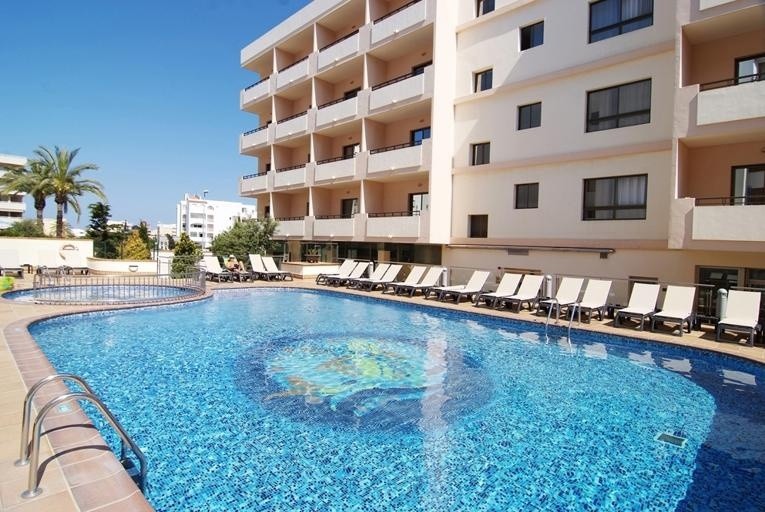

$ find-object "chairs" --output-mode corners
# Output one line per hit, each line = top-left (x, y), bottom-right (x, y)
(475, 272), (523, 309)
(246, 252), (294, 283)
(381, 265), (427, 295)
(713, 290), (764, 349)
(201, 256), (254, 283)
(499, 274), (545, 312)
(0, 251), (91, 278)
(567, 279), (614, 325)
(614, 282), (660, 332)
(314, 259), (403, 292)
(651, 283), (699, 337)
(537, 276), (585, 320)
(438, 270), (492, 306)
(393, 267), (447, 299)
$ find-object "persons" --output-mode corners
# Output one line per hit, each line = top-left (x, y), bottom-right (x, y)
(225, 254), (245, 274)
